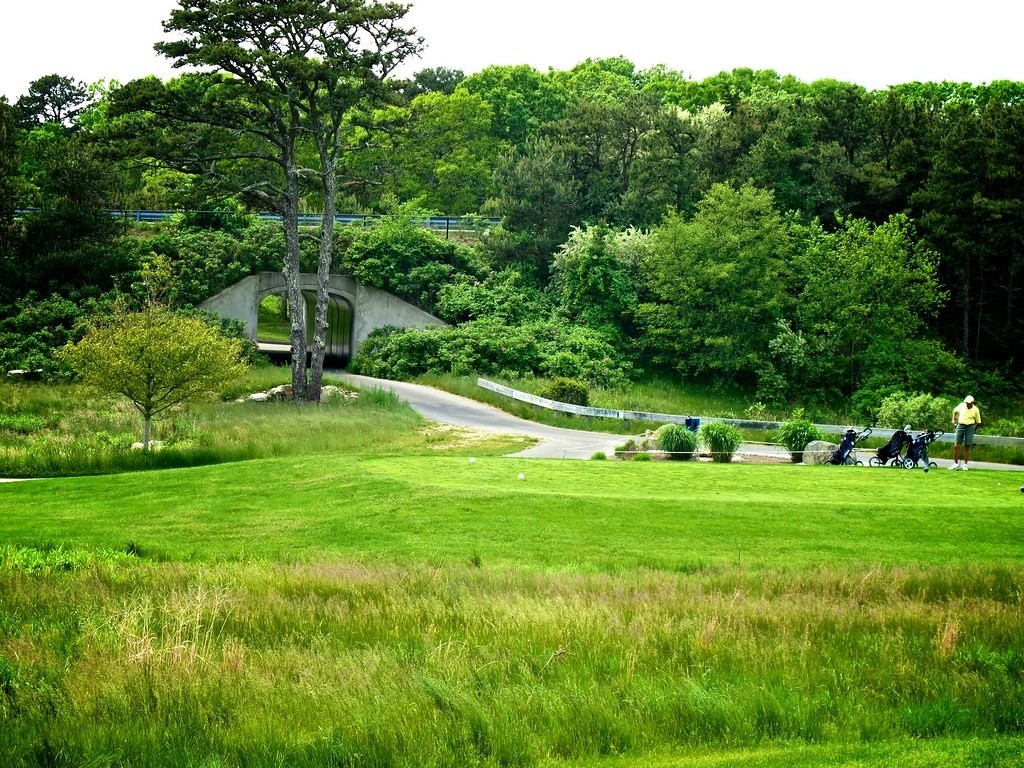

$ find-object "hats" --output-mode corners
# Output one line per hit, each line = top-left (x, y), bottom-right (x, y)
(964, 396), (974, 403)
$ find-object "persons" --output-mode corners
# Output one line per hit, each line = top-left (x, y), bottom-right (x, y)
(948, 396), (981, 471)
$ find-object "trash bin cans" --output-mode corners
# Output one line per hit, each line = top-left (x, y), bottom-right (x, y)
(685, 418), (700, 432)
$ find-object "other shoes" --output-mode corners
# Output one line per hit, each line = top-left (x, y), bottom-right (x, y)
(948, 463), (959, 471)
(962, 464), (968, 471)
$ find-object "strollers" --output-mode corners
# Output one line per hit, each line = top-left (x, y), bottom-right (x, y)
(901, 429), (945, 467)
(823, 428), (873, 466)
(869, 429), (914, 470)
